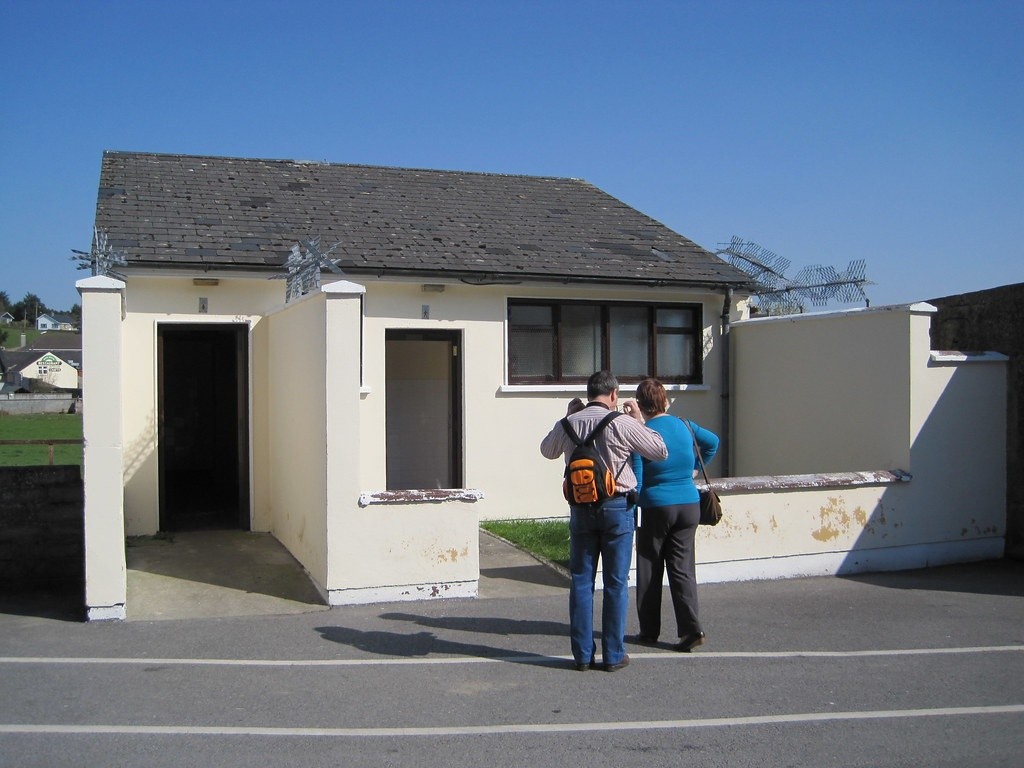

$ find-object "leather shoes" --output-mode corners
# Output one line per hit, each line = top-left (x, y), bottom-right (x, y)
(638, 633), (657, 643)
(672, 630), (705, 652)
(603, 655), (629, 672)
(577, 657), (595, 671)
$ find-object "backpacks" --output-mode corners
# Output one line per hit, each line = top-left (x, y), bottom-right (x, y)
(560, 411), (632, 508)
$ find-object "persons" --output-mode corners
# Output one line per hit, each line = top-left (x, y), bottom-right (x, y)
(540, 370), (669, 673)
(627, 378), (720, 652)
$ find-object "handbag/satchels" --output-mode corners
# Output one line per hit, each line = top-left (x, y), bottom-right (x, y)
(698, 488), (723, 526)
